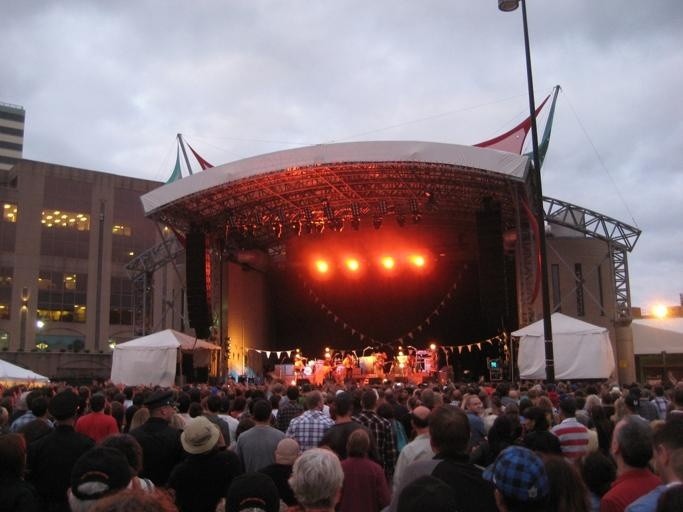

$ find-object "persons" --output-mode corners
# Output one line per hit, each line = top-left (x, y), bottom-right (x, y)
(585, 395), (600, 410)
(347, 375), (484, 404)
(285, 391), (335, 451)
(589, 405), (612, 451)
(258, 439), (301, 506)
(497, 384), (520, 407)
(518, 406), (563, 458)
(483, 392), (505, 433)
(337, 429), (390, 512)
(319, 392), (379, 460)
(476, 415), (524, 467)
(0, 433), (39, 512)
(480, 446), (550, 511)
(602, 417), (662, 512)
(66, 441), (134, 512)
(542, 455), (592, 512)
(355, 390), (397, 481)
(276, 386), (304, 430)
(611, 396), (646, 463)
(149, 384), (194, 392)
(236, 389), (278, 436)
(236, 418), (256, 440)
(165, 415), (233, 512)
(537, 397), (557, 428)
(519, 395), (535, 414)
(0, 381), (20, 434)
(27, 390), (93, 512)
(670, 382), (683, 421)
(76, 394), (118, 442)
(188, 402), (204, 419)
(376, 475), (459, 512)
(579, 453), (617, 496)
(10, 393), (54, 433)
(104, 434), (158, 495)
(461, 394), (493, 451)
(622, 421), (683, 512)
(551, 399), (589, 465)
(129, 390), (187, 476)
(502, 403), (521, 419)
(204, 396), (231, 448)
(190, 376), (346, 400)
(235, 401), (292, 472)
(289, 448), (344, 511)
(601, 383), (648, 400)
(401, 397), (424, 438)
(104, 382), (149, 431)
(390, 405), (433, 507)
(379, 402), (407, 454)
(649, 384), (670, 421)
(174, 395), (192, 423)
(393, 404), (496, 511)
(20, 398), (51, 440)
(520, 379), (601, 398)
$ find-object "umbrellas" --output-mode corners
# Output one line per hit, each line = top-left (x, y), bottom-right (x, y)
(0, 358), (49, 381)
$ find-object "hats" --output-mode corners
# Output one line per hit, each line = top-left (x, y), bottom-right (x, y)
(625, 392), (639, 408)
(70, 432), (144, 500)
(180, 416), (221, 456)
(483, 446), (552, 502)
(143, 389), (182, 409)
(48, 389), (79, 418)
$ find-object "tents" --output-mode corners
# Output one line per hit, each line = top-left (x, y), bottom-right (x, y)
(109, 329), (221, 388)
(512, 313), (615, 380)
